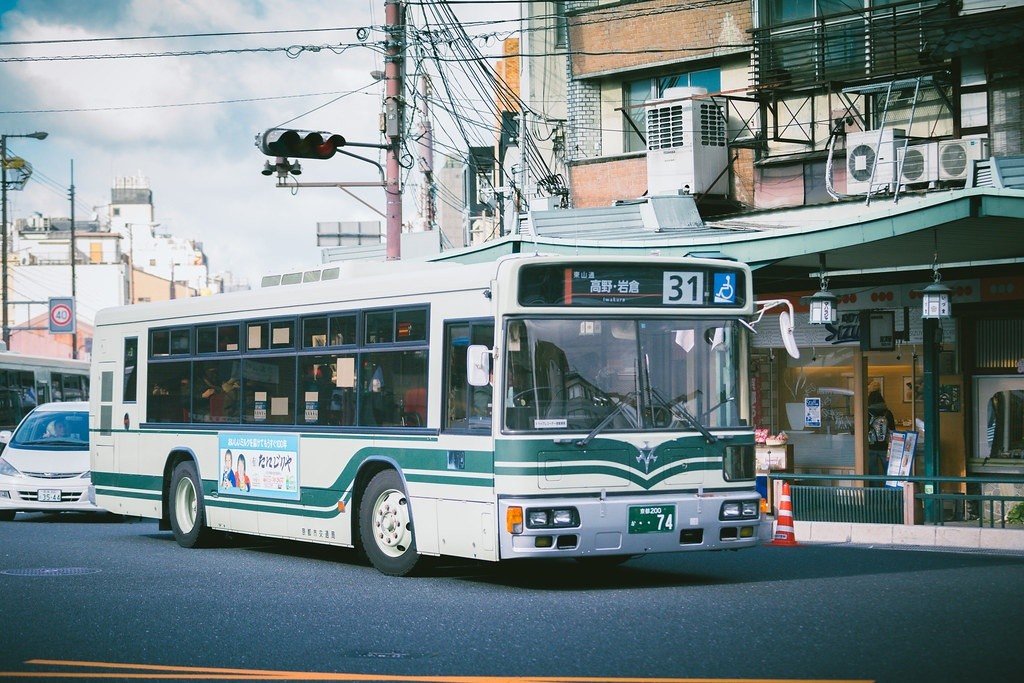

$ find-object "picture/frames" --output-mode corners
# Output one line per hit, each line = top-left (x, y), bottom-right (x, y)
(902, 375), (925, 403)
(846, 376), (883, 417)
(937, 383), (961, 415)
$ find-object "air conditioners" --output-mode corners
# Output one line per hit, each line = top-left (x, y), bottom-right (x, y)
(846, 128), (906, 197)
(939, 138), (991, 181)
(895, 142), (939, 192)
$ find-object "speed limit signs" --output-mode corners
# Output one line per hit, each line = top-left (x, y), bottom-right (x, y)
(52, 304), (72, 326)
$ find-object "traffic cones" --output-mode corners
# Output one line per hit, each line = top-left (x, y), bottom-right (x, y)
(763, 482), (807, 547)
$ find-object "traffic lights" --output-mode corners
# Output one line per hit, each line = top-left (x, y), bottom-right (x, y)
(261, 128), (347, 159)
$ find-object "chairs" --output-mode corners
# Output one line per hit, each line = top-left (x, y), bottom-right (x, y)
(403, 389), (427, 428)
(210, 393), (226, 423)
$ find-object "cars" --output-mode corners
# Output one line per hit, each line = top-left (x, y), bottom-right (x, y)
(0, 401), (110, 521)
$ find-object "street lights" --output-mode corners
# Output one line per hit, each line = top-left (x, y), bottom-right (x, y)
(1, 131), (49, 350)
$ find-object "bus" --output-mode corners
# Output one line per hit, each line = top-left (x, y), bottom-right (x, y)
(88, 254), (801, 576)
(0, 351), (91, 455)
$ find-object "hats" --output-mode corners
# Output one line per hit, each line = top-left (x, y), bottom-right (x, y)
(868, 381), (880, 392)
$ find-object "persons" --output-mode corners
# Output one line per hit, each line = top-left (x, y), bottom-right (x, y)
(223, 450), (250, 492)
(471, 362), (534, 418)
(865, 381), (895, 487)
(196, 360), (240, 415)
(43, 419), (68, 437)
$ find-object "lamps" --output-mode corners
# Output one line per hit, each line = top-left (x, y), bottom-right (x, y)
(800, 252), (839, 325)
(913, 231), (955, 320)
